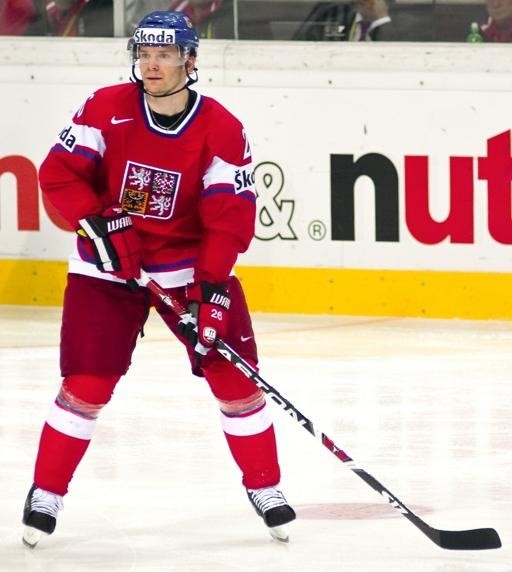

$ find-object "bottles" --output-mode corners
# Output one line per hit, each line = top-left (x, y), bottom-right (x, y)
(463, 21), (483, 43)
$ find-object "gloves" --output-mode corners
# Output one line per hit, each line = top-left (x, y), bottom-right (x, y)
(76, 204), (142, 281)
(177, 283), (230, 360)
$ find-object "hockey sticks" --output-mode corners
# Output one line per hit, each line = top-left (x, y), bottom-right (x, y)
(135, 268), (502, 550)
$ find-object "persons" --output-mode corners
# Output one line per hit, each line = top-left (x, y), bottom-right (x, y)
(165, 0), (224, 26)
(0, 1), (109, 36)
(18, 13), (296, 536)
(346, 0), (430, 41)
(474, 0), (511, 43)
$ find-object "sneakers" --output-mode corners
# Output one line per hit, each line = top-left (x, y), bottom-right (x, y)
(22, 484), (60, 534)
(247, 485), (296, 528)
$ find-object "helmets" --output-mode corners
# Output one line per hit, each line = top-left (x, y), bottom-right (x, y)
(130, 10), (198, 48)
